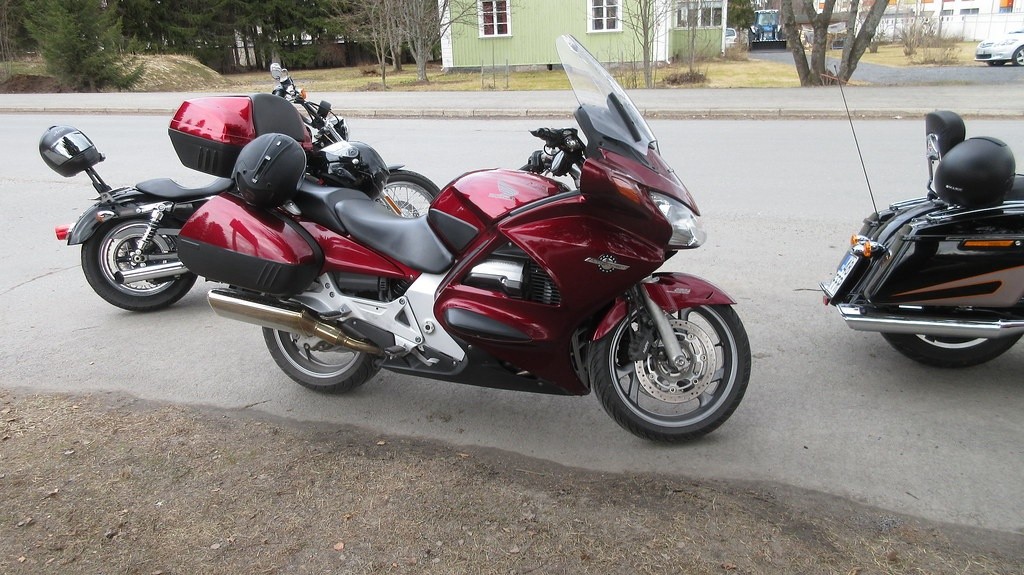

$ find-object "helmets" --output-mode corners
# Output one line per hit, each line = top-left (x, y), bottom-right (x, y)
(315, 139), (392, 200)
(935, 137), (1017, 210)
(231, 131), (307, 209)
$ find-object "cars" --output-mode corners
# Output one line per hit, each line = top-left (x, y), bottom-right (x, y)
(747, 9), (788, 51)
(725, 27), (738, 49)
(973, 26), (1024, 66)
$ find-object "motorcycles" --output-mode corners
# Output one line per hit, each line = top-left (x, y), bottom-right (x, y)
(818, 64), (1024, 369)
(174, 32), (753, 445)
(33, 59), (444, 313)
